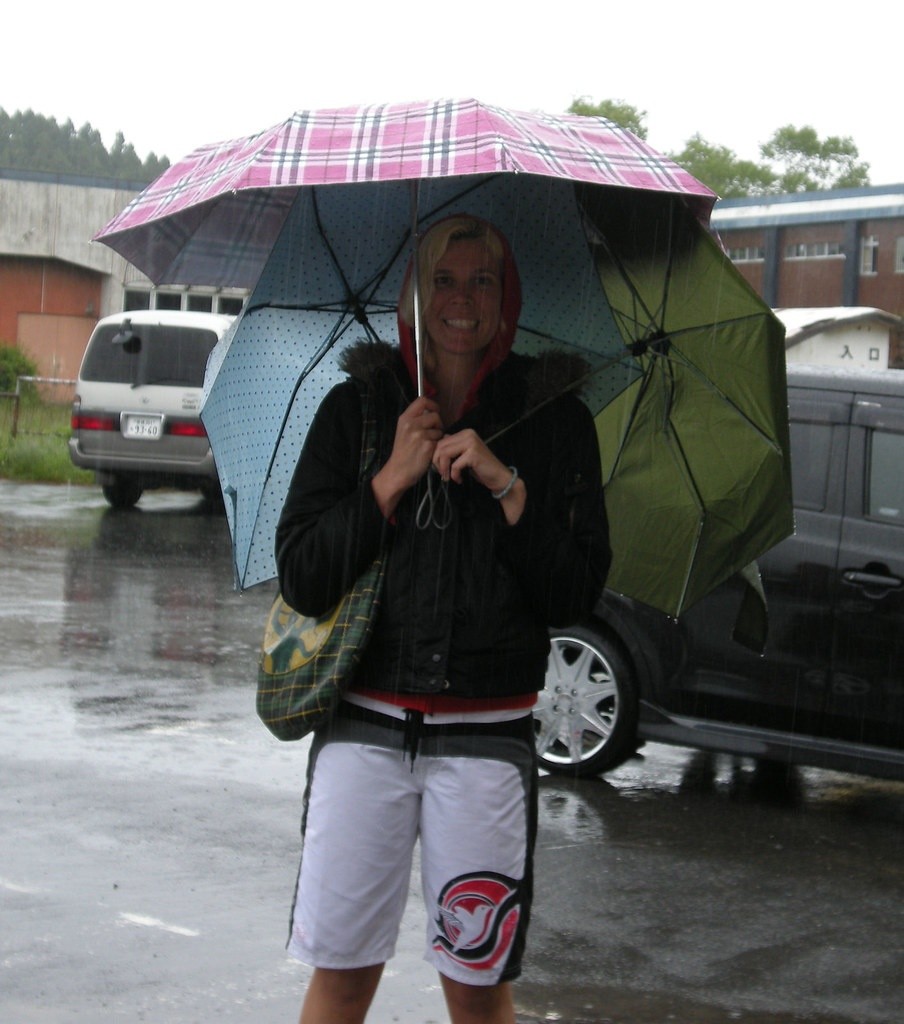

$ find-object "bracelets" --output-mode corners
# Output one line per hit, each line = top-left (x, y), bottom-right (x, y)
(492, 466), (517, 499)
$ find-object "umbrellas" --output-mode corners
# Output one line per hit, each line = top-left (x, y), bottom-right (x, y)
(588, 190), (795, 624)
(90, 97), (719, 294)
(199, 170), (646, 599)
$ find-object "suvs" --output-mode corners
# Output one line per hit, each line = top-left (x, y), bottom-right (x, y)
(535, 367), (903, 779)
(67, 308), (240, 510)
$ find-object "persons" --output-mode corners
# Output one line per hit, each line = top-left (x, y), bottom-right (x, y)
(274, 213), (612, 1024)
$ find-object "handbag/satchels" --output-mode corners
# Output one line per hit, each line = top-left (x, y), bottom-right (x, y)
(256, 377), (393, 740)
